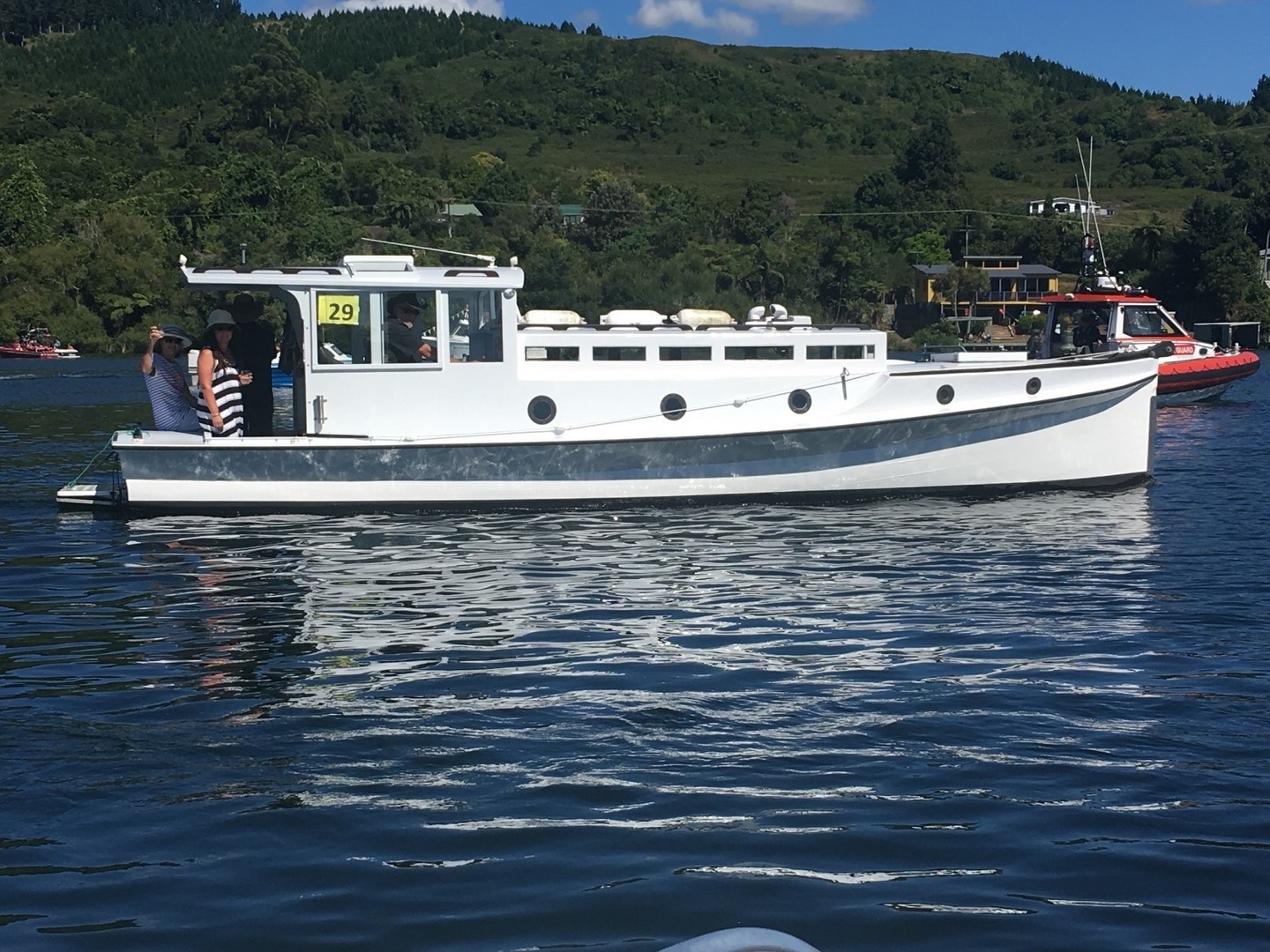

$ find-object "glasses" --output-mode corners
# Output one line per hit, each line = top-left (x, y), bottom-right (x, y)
(213, 324), (234, 331)
(397, 306), (420, 315)
(164, 336), (183, 345)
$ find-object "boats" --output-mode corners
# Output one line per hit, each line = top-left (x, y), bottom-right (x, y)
(187, 343), (351, 388)
(0, 333), (81, 359)
(925, 137), (1261, 407)
(56, 237), (1159, 513)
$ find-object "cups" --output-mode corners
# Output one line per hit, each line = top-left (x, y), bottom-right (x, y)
(156, 323), (165, 339)
(241, 370), (250, 384)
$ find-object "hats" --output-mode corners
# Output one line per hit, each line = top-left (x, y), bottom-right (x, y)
(205, 309), (236, 336)
(1081, 312), (1097, 321)
(387, 292), (428, 314)
(156, 322), (192, 350)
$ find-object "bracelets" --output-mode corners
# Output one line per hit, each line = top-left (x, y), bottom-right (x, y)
(212, 413), (220, 417)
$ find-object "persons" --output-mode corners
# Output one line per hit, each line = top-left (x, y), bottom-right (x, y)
(231, 292), (278, 435)
(52, 337), (61, 349)
(978, 327), (991, 343)
(1072, 314), (1100, 353)
(386, 291), (462, 362)
(67, 341), (74, 349)
(13, 341), (23, 351)
(29, 338), (39, 347)
(197, 309), (254, 437)
(141, 325), (201, 431)
(1027, 322), (1045, 360)
